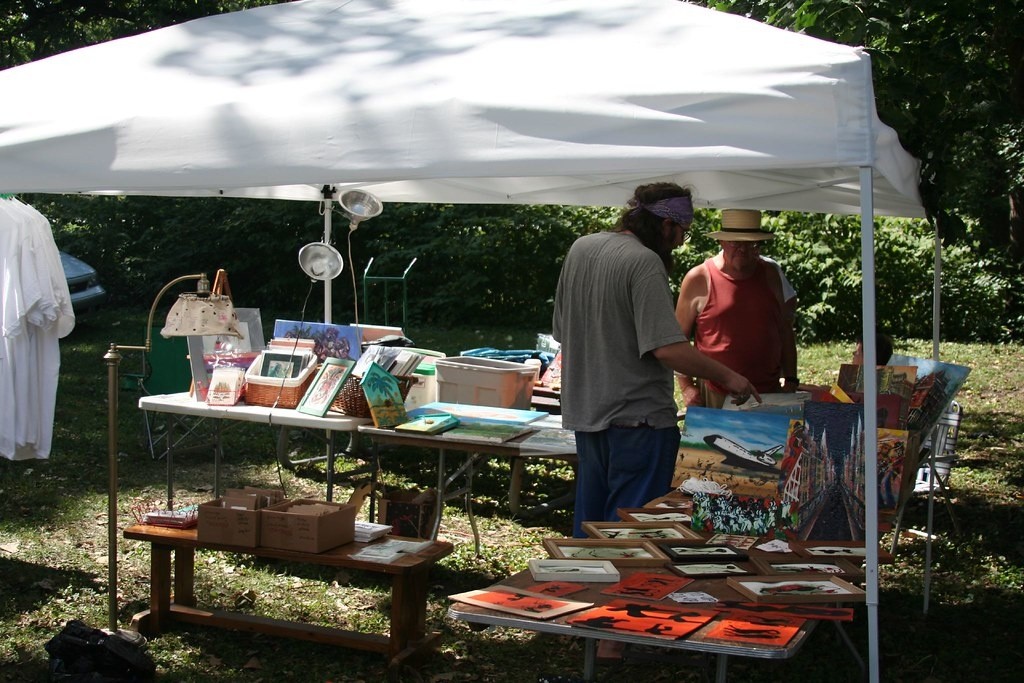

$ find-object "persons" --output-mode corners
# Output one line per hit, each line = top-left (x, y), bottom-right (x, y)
(797, 334), (893, 393)
(675, 209), (797, 409)
(552, 183), (762, 538)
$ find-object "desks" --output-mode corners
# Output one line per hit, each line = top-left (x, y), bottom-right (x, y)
(135, 390), (419, 506)
(357, 410), (583, 553)
(450, 489), (825, 683)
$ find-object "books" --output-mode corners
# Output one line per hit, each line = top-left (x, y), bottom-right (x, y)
(442, 422), (533, 443)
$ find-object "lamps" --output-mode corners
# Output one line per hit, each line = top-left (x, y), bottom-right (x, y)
(93, 273), (244, 656)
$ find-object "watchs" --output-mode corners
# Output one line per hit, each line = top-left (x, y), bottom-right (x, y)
(785, 377), (799, 387)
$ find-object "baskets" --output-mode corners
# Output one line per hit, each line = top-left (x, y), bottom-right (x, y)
(244, 354), (318, 408)
(329, 375), (418, 418)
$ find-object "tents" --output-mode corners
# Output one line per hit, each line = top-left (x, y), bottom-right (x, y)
(0, 0), (941, 682)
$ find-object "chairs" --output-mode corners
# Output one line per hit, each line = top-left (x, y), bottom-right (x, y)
(909, 402), (964, 500)
(132, 324), (227, 464)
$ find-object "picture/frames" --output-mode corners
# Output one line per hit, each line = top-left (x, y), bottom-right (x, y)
(529, 503), (897, 609)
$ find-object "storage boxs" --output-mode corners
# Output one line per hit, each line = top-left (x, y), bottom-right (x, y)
(435, 356), (539, 412)
(378, 498), (437, 541)
(197, 498), (289, 547)
(261, 498), (357, 554)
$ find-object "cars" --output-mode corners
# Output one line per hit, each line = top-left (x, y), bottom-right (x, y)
(58, 251), (107, 324)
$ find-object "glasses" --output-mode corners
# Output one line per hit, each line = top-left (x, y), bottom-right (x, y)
(678, 223), (691, 241)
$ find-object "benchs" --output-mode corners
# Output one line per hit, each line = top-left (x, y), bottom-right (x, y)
(122, 524), (456, 672)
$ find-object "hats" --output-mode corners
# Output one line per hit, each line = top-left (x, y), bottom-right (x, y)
(702, 209), (773, 241)
